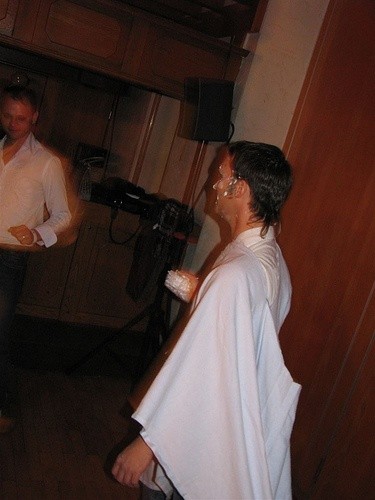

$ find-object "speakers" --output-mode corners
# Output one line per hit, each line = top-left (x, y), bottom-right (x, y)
(178, 76), (234, 142)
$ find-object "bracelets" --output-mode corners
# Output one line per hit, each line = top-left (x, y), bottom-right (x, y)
(30, 229), (37, 243)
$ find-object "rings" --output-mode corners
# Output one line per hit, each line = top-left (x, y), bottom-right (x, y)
(23, 236), (25, 238)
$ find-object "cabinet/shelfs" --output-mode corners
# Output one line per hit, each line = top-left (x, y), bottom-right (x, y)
(0, 0), (250, 330)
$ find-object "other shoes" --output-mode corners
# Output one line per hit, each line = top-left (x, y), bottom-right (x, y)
(0, 416), (17, 433)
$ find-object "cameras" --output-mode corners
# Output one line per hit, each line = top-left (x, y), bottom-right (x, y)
(89, 177), (194, 237)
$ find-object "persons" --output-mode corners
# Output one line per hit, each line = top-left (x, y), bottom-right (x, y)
(0, 86), (72, 431)
(112, 139), (301, 500)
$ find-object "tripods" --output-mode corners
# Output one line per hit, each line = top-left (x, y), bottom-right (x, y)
(66, 222), (194, 395)
